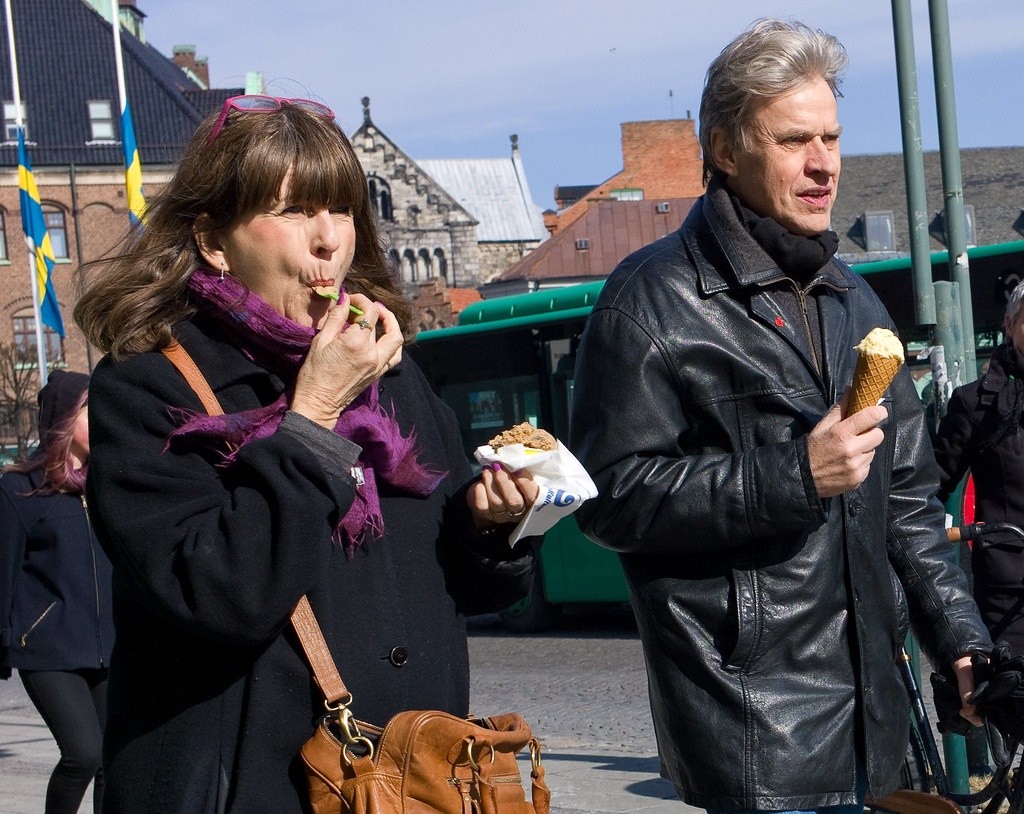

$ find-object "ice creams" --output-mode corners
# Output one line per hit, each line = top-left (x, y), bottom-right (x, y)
(488, 421), (556, 453)
(845, 327), (905, 419)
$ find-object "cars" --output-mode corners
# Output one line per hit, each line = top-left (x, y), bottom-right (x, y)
(0, 443), (39, 468)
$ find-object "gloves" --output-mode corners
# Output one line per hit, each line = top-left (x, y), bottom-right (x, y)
(930, 639), (1023, 768)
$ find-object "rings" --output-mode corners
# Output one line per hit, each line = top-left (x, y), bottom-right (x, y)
(491, 509), (506, 515)
(506, 506), (526, 516)
(386, 362), (392, 370)
(354, 319), (373, 331)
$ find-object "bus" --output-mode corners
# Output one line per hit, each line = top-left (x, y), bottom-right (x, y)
(405, 235), (1023, 634)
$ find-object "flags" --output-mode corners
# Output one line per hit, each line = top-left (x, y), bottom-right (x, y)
(121, 99), (148, 236)
(18, 127), (65, 339)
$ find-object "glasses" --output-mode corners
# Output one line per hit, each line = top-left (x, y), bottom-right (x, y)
(206, 94), (335, 147)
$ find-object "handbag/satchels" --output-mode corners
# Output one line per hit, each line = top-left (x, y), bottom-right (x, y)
(299, 709), (551, 814)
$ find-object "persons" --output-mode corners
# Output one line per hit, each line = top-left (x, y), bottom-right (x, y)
(72, 104), (539, 814)
(569, 20), (994, 814)
(934, 281), (1024, 656)
(0, 368), (110, 814)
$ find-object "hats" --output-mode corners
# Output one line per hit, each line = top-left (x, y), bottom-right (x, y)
(37, 370), (90, 444)
